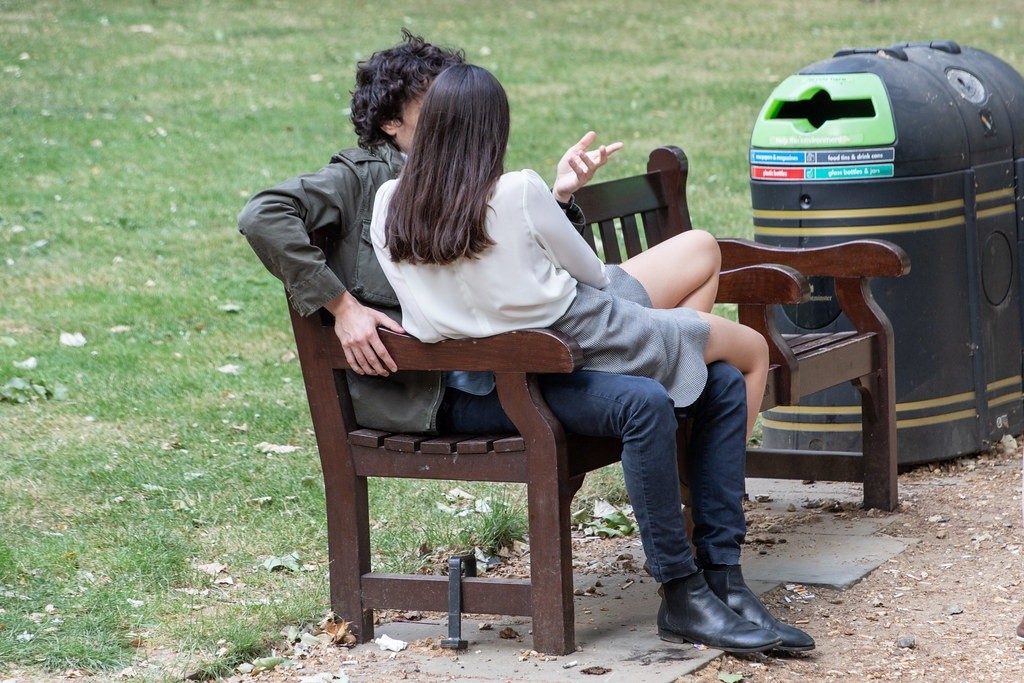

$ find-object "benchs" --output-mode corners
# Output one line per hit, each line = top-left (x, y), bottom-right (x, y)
(286, 146), (911, 656)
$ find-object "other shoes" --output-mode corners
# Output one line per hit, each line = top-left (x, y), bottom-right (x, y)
(643, 479), (698, 577)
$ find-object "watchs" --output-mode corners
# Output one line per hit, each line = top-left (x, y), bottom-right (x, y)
(555, 193), (575, 209)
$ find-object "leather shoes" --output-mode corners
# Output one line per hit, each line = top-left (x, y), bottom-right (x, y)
(657, 563), (783, 653)
(703, 564), (815, 651)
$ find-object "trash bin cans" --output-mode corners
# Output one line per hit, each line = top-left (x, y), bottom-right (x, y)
(748, 41), (1024, 464)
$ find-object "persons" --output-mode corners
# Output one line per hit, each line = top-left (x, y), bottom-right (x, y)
(238, 31), (816, 654)
